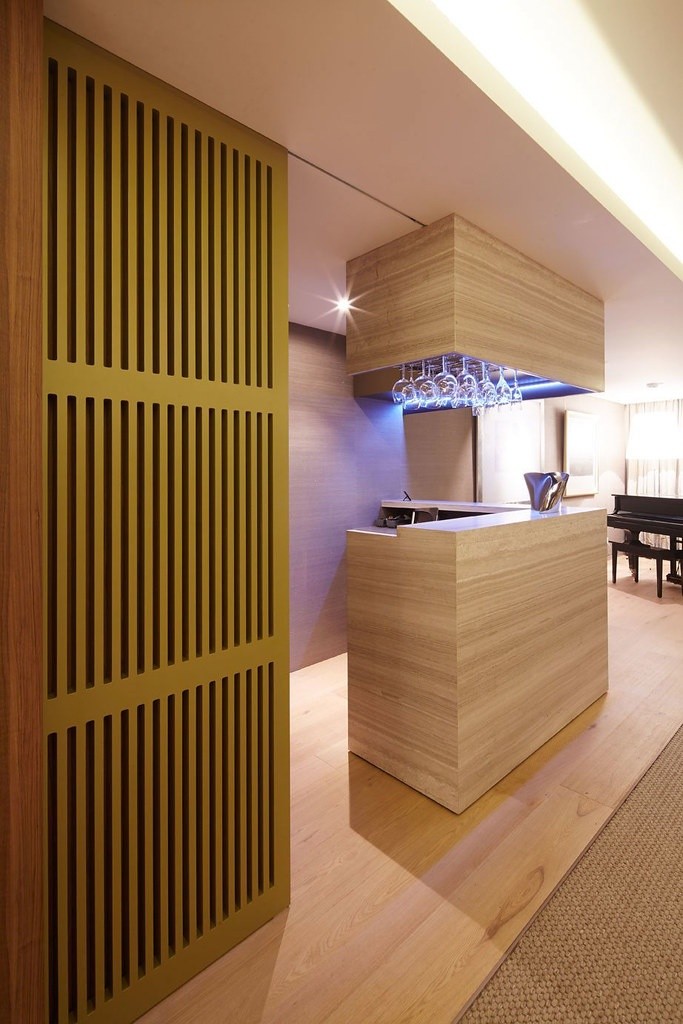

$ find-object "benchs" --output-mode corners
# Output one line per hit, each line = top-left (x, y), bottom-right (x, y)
(610, 540), (683, 599)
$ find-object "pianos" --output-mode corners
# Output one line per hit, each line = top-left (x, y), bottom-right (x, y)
(607, 494), (683, 598)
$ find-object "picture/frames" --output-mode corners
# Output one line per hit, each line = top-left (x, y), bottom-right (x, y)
(563, 409), (599, 498)
(474, 399), (547, 505)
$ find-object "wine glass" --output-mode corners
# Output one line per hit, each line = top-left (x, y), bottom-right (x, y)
(390, 354), (523, 417)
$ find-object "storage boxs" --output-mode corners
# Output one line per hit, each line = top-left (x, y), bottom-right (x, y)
(376, 518), (387, 528)
(386, 519), (411, 529)
